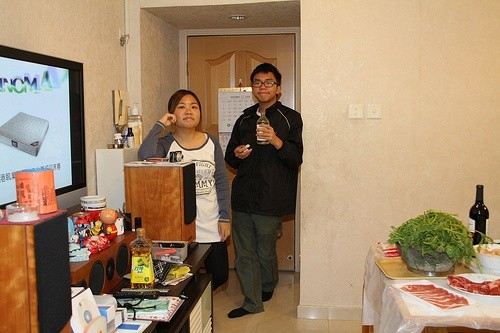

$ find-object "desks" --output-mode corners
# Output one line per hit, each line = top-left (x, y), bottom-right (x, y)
(363, 244), (500, 333)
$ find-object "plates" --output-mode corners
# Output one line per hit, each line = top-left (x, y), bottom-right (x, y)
(375, 256), (485, 280)
(448, 273), (500, 298)
(392, 280), (478, 312)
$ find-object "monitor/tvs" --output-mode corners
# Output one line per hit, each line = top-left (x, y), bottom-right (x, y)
(0, 44), (87, 208)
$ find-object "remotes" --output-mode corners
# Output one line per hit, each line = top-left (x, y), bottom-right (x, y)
(113, 291), (160, 300)
(121, 288), (170, 295)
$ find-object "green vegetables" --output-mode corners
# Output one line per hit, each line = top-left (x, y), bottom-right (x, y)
(387, 211), (500, 274)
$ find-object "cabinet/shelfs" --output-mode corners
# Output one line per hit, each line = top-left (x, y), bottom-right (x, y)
(142, 244), (213, 333)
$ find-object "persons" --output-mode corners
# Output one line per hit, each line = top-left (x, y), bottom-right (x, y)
(138, 89), (231, 291)
(223, 62), (303, 319)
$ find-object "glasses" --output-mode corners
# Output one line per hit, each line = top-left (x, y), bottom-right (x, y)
(252, 79), (279, 88)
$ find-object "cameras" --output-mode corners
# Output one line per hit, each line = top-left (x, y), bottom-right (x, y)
(170, 150), (184, 162)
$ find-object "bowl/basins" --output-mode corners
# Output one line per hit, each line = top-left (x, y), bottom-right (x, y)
(396, 241), (458, 277)
(5, 202), (40, 223)
(113, 143), (123, 148)
(79, 195), (107, 211)
(472, 243), (500, 276)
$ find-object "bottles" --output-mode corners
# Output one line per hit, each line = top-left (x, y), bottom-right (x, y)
(468, 185), (490, 258)
(114, 133), (124, 144)
(256, 113), (270, 144)
(129, 227), (155, 290)
(126, 128), (135, 147)
